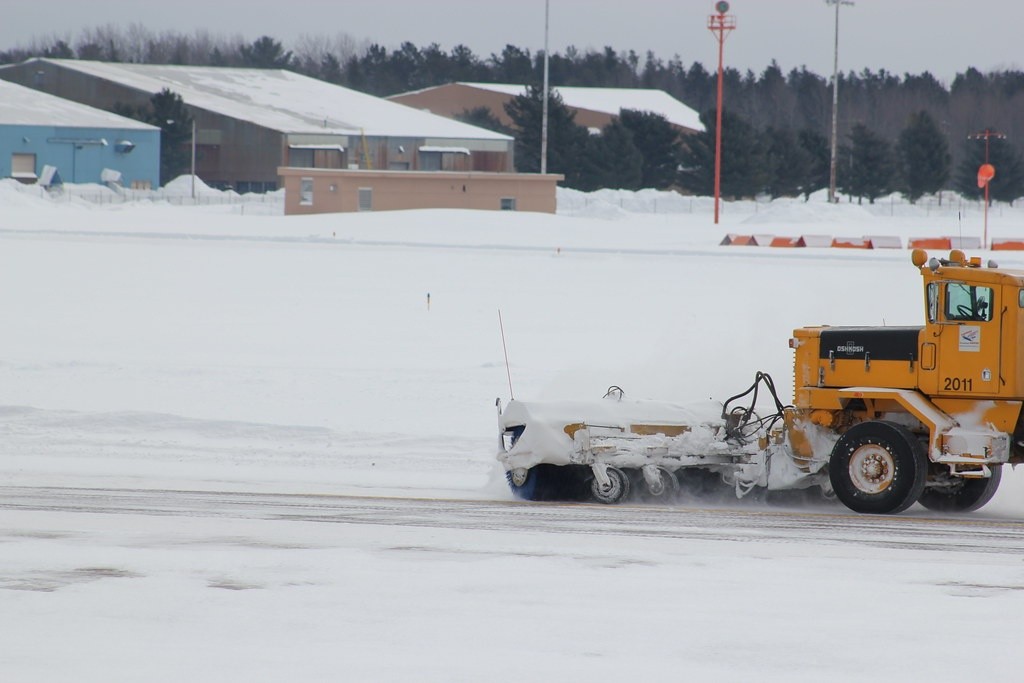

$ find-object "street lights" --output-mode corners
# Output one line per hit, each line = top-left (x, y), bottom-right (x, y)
(966, 130), (1007, 249)
(825, 0), (854, 203)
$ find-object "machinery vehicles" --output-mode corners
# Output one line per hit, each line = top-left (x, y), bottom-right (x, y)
(495, 250), (1024, 514)
